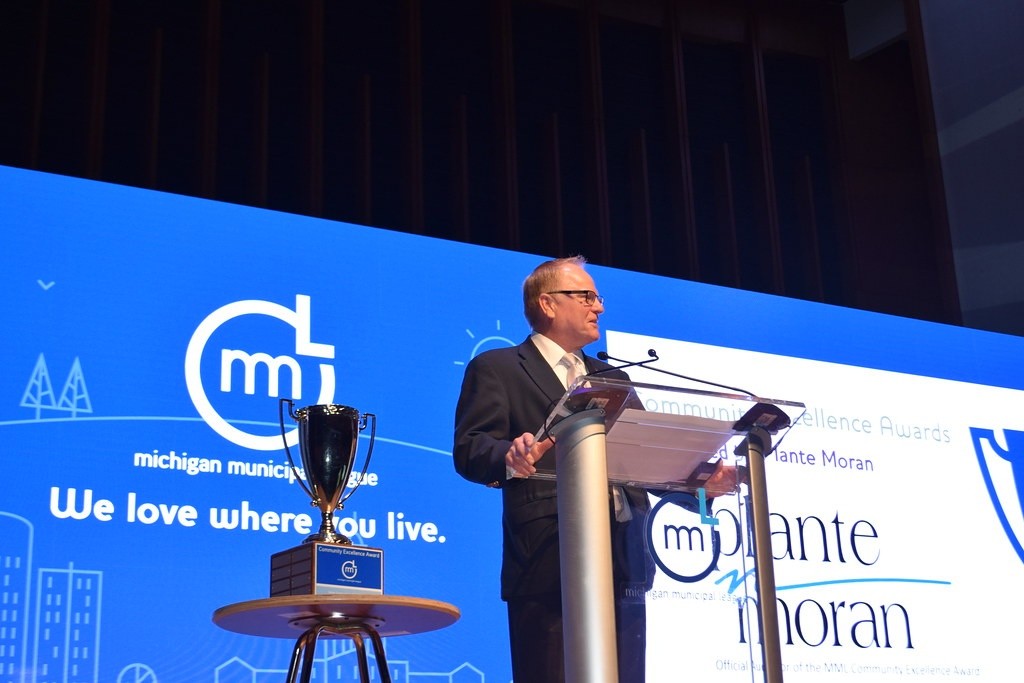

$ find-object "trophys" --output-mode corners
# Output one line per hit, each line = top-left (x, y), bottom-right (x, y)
(279, 398), (376, 545)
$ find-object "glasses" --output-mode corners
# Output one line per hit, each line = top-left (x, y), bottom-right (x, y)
(538, 290), (604, 306)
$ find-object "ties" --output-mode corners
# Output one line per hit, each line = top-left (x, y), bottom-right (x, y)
(562, 353), (624, 518)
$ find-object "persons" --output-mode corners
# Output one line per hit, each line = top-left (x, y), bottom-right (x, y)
(454, 254), (750, 683)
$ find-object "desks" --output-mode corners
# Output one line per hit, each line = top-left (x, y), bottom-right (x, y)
(212, 593), (461, 683)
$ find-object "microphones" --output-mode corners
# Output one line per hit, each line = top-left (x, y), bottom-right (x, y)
(563, 348), (791, 436)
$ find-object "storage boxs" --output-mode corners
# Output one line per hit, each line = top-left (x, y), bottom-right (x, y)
(269, 542), (384, 597)
